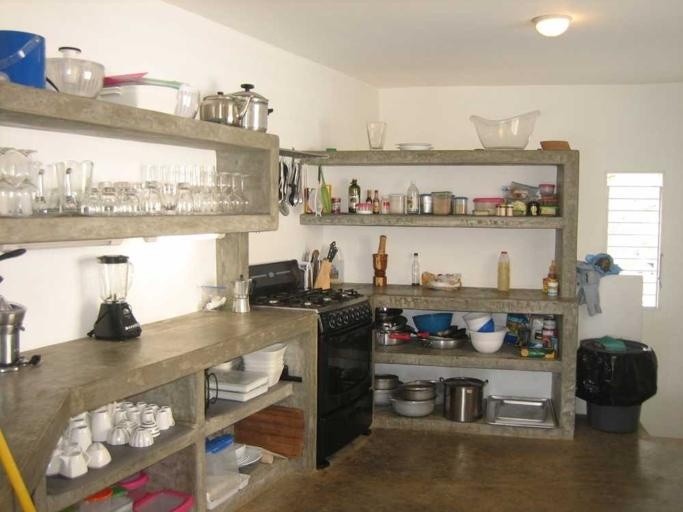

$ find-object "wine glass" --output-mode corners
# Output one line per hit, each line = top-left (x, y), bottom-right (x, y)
(0, 146), (43, 218)
(141, 165), (251, 215)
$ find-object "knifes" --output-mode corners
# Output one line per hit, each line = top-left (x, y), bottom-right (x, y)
(326, 240), (338, 261)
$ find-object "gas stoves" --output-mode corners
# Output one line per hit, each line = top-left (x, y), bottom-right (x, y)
(245, 285), (373, 331)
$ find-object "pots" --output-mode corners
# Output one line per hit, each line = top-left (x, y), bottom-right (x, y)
(416, 326), (467, 351)
(438, 374), (489, 422)
(371, 374), (437, 419)
(0, 302), (31, 369)
(230, 81), (275, 135)
(375, 307), (412, 347)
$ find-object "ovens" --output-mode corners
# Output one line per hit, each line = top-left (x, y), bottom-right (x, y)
(317, 325), (373, 470)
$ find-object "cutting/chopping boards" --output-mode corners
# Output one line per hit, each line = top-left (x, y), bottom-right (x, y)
(232, 405), (305, 461)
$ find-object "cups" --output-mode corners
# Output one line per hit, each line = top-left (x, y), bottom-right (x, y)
(44, 405), (111, 478)
(366, 122), (387, 150)
(45, 161), (142, 216)
(112, 401), (176, 450)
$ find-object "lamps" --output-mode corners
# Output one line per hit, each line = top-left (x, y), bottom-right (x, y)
(532, 13), (572, 37)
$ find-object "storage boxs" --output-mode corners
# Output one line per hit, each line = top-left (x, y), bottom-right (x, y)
(97, 77), (180, 114)
(204, 433), (239, 501)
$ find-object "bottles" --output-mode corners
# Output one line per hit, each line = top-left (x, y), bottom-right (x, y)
(521, 349), (549, 358)
(496, 251), (509, 293)
(347, 178), (361, 213)
(496, 204), (514, 217)
(406, 179), (419, 213)
(542, 260), (558, 298)
(410, 251), (420, 288)
(366, 189), (390, 215)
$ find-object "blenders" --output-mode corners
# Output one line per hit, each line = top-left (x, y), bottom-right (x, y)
(85, 254), (142, 341)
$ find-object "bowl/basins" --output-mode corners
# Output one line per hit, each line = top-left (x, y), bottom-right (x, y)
(210, 340), (288, 386)
(410, 311), (454, 335)
(467, 324), (508, 354)
(46, 57), (107, 97)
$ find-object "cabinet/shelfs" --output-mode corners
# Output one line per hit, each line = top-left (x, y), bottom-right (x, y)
(0, 79), (318, 512)
(300, 150), (580, 440)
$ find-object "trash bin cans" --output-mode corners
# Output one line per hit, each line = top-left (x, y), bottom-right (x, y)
(578, 337), (653, 433)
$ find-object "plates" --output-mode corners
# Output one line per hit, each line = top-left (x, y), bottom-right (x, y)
(226, 443), (262, 468)
(395, 143), (435, 150)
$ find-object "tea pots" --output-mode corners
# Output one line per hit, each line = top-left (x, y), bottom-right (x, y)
(229, 276), (252, 314)
(196, 90), (251, 125)
